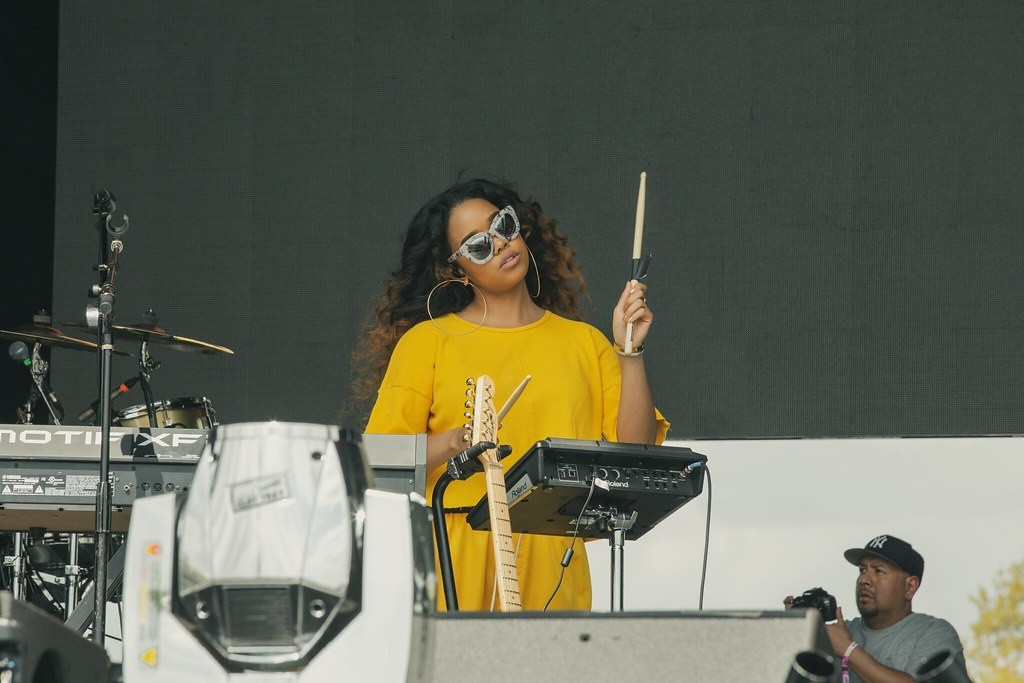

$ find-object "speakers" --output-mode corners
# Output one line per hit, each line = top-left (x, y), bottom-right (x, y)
(0, 590), (112, 683)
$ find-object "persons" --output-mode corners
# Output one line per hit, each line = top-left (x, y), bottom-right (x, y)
(356, 180), (670, 612)
(783, 535), (966, 683)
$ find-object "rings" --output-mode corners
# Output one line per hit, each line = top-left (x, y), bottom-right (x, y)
(643, 296), (647, 303)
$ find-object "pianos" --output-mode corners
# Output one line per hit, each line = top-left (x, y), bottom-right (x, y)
(0, 421), (212, 534)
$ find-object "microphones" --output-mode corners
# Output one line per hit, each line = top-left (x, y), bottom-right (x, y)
(9, 341), (66, 416)
(78, 378), (139, 422)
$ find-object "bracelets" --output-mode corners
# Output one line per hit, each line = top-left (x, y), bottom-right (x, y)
(614, 343), (645, 357)
(842, 640), (858, 682)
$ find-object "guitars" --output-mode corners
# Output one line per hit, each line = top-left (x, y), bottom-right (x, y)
(460, 370), (523, 613)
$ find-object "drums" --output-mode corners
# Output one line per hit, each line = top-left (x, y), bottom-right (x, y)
(115, 396), (221, 430)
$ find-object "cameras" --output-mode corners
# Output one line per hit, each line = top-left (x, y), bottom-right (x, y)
(790, 587), (837, 623)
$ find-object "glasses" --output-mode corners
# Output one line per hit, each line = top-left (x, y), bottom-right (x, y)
(445, 204), (521, 265)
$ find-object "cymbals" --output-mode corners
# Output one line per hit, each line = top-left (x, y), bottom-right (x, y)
(62, 318), (234, 358)
(0, 322), (136, 358)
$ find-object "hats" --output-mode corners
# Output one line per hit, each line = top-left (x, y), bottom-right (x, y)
(844, 534), (925, 578)
